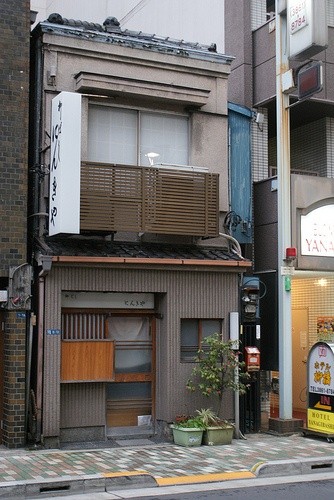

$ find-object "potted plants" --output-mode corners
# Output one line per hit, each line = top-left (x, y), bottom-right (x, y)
(168, 417), (206, 448)
(186, 332), (250, 445)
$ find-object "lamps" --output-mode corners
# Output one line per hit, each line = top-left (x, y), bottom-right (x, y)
(287, 247), (296, 260)
